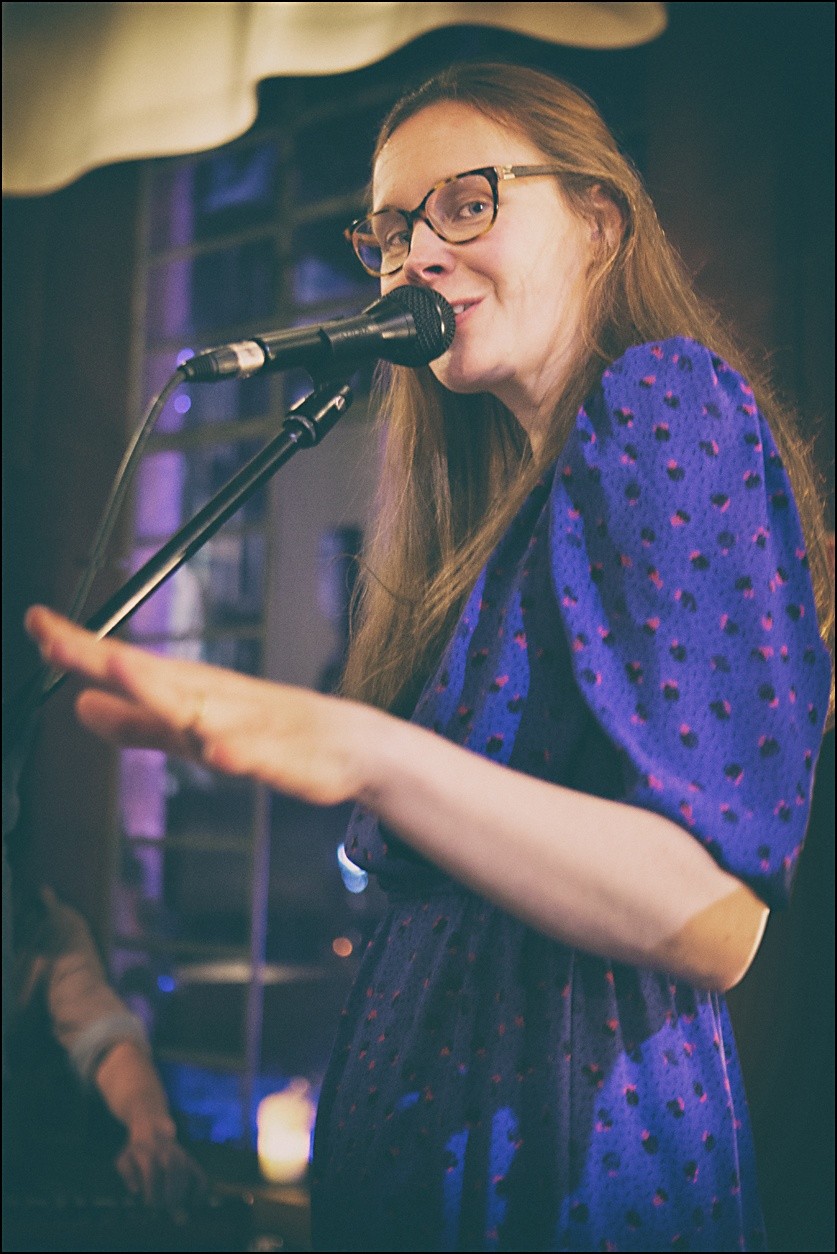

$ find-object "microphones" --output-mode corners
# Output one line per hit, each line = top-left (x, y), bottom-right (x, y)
(180, 285), (456, 382)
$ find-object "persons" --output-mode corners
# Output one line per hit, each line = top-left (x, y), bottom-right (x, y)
(1, 872), (208, 1226)
(25, 62), (836, 1254)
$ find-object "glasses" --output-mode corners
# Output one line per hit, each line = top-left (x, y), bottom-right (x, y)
(345, 163), (565, 276)
